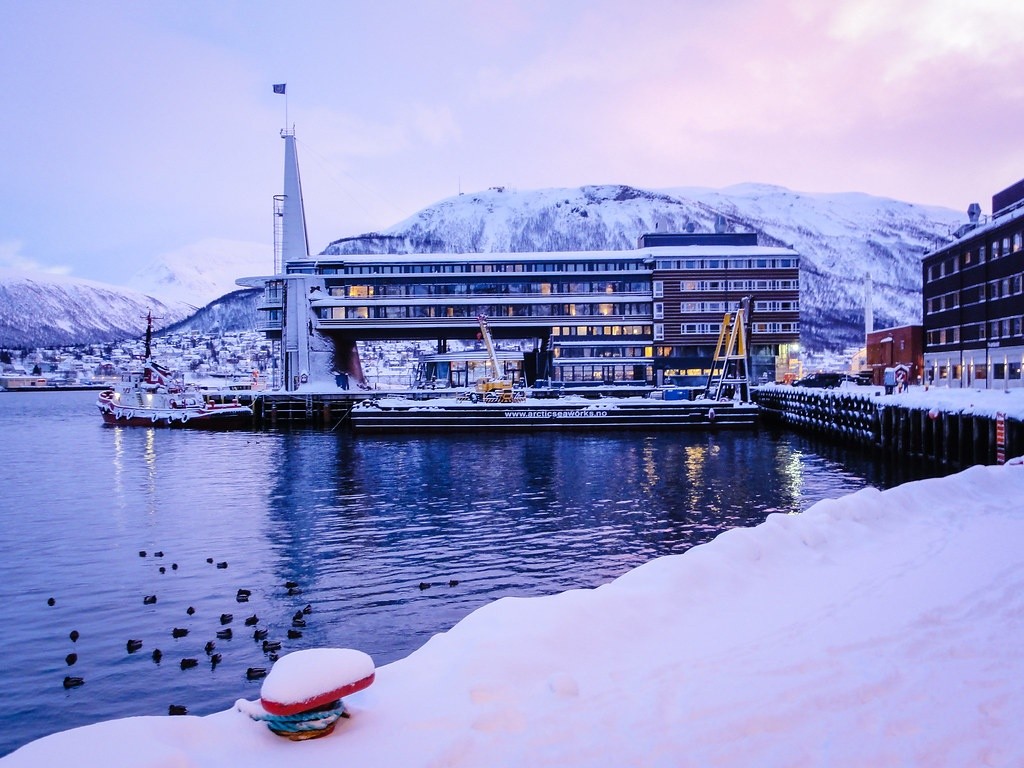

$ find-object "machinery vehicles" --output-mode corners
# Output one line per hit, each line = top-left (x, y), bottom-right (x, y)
(469, 311), (527, 404)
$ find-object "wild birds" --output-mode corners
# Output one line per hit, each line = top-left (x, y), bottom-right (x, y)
(45, 548), (461, 715)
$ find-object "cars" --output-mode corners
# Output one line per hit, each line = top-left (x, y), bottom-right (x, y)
(792, 372), (842, 390)
(856, 376), (871, 385)
(840, 373), (859, 383)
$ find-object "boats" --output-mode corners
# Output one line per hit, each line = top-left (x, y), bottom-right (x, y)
(349, 310), (761, 435)
(95, 309), (254, 430)
